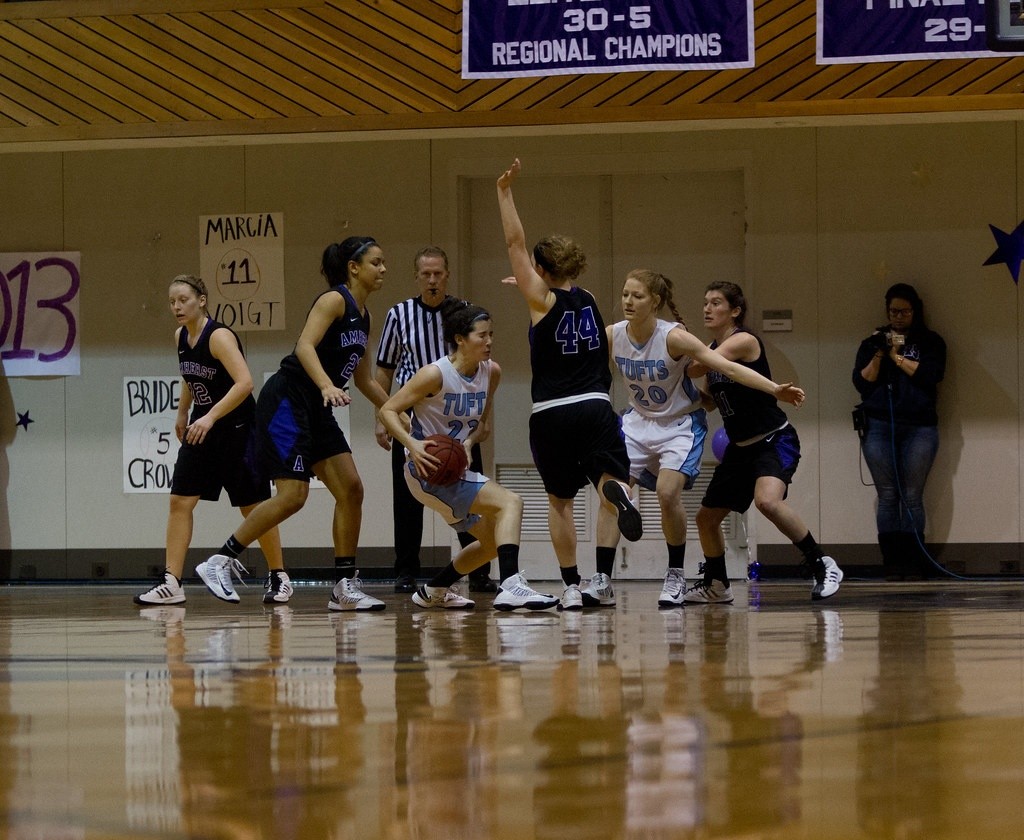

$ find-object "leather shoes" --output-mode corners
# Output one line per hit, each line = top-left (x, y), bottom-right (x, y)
(468, 575), (497, 592)
(395, 575), (417, 592)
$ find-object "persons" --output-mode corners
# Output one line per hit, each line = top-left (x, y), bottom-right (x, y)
(132, 276), (295, 605)
(852, 283), (946, 582)
(580, 269), (805, 602)
(496, 157), (642, 610)
(683, 281), (844, 604)
(375, 247), (498, 595)
(195, 237), (412, 612)
(379, 297), (561, 612)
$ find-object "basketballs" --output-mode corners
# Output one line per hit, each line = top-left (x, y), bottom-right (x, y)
(417, 433), (468, 486)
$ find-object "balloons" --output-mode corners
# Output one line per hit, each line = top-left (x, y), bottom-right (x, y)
(711, 427), (729, 462)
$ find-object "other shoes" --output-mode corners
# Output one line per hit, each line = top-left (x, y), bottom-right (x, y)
(886, 568), (903, 581)
(904, 568), (920, 580)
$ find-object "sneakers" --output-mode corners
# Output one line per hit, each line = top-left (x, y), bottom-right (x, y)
(493, 570), (560, 611)
(581, 572), (616, 607)
(584, 609), (615, 633)
(557, 575), (583, 609)
(133, 566), (187, 606)
(329, 611), (386, 630)
(658, 606), (687, 630)
(807, 610), (844, 674)
(602, 479), (644, 542)
(412, 610), (476, 633)
(263, 604), (294, 630)
(327, 570), (386, 611)
(684, 602), (734, 630)
(559, 609), (583, 632)
(684, 578), (734, 603)
(493, 611), (560, 647)
(657, 568), (688, 606)
(194, 554), (250, 604)
(261, 570), (293, 602)
(140, 604), (186, 637)
(800, 555), (844, 599)
(412, 584), (475, 609)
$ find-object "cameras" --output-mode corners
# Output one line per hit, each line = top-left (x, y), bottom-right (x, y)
(871, 325), (893, 352)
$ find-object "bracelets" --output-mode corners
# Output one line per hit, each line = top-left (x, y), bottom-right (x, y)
(896, 355), (904, 365)
(876, 355), (883, 357)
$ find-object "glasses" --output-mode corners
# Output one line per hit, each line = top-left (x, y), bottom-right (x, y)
(887, 307), (913, 317)
